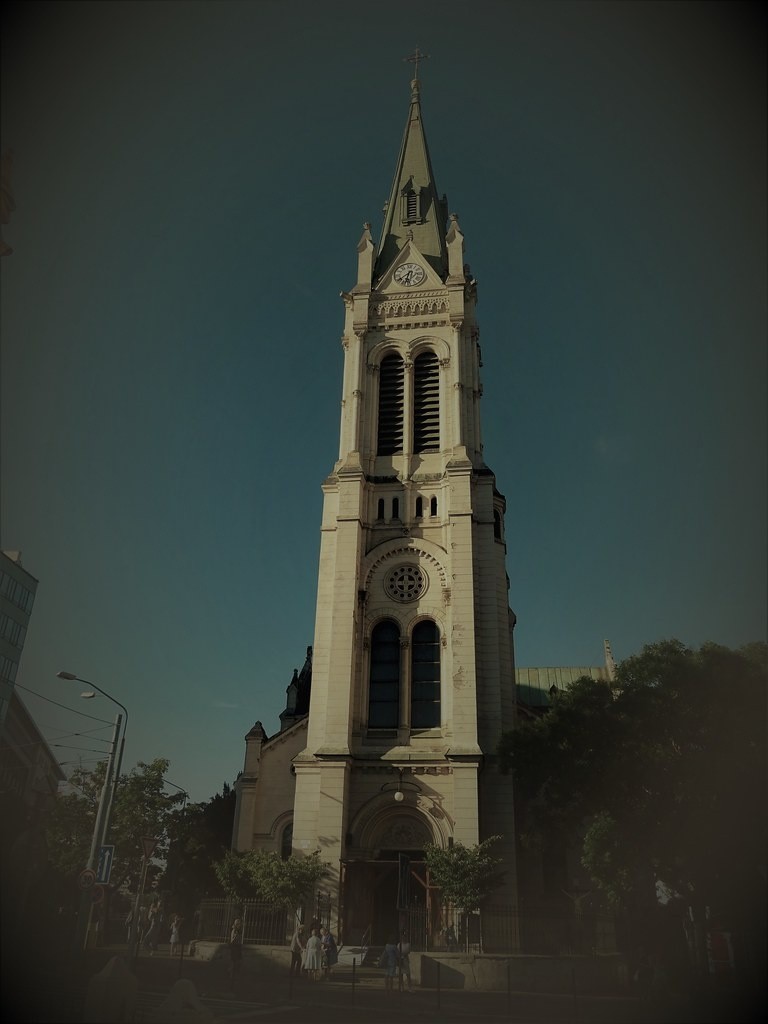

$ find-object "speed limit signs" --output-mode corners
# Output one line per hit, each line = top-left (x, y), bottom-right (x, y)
(76, 869), (97, 889)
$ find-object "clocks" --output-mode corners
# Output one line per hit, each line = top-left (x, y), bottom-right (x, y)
(394, 262), (426, 287)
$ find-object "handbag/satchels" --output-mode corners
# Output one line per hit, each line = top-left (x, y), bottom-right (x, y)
(379, 950), (388, 969)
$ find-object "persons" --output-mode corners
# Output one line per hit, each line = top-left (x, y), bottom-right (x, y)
(397, 935), (416, 994)
(227, 919), (243, 979)
(304, 929), (322, 984)
(125, 900), (167, 957)
(319, 928), (338, 981)
(289, 925), (305, 977)
(378, 936), (400, 991)
(170, 915), (186, 957)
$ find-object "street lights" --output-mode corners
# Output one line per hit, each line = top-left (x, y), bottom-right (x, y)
(55, 669), (129, 949)
